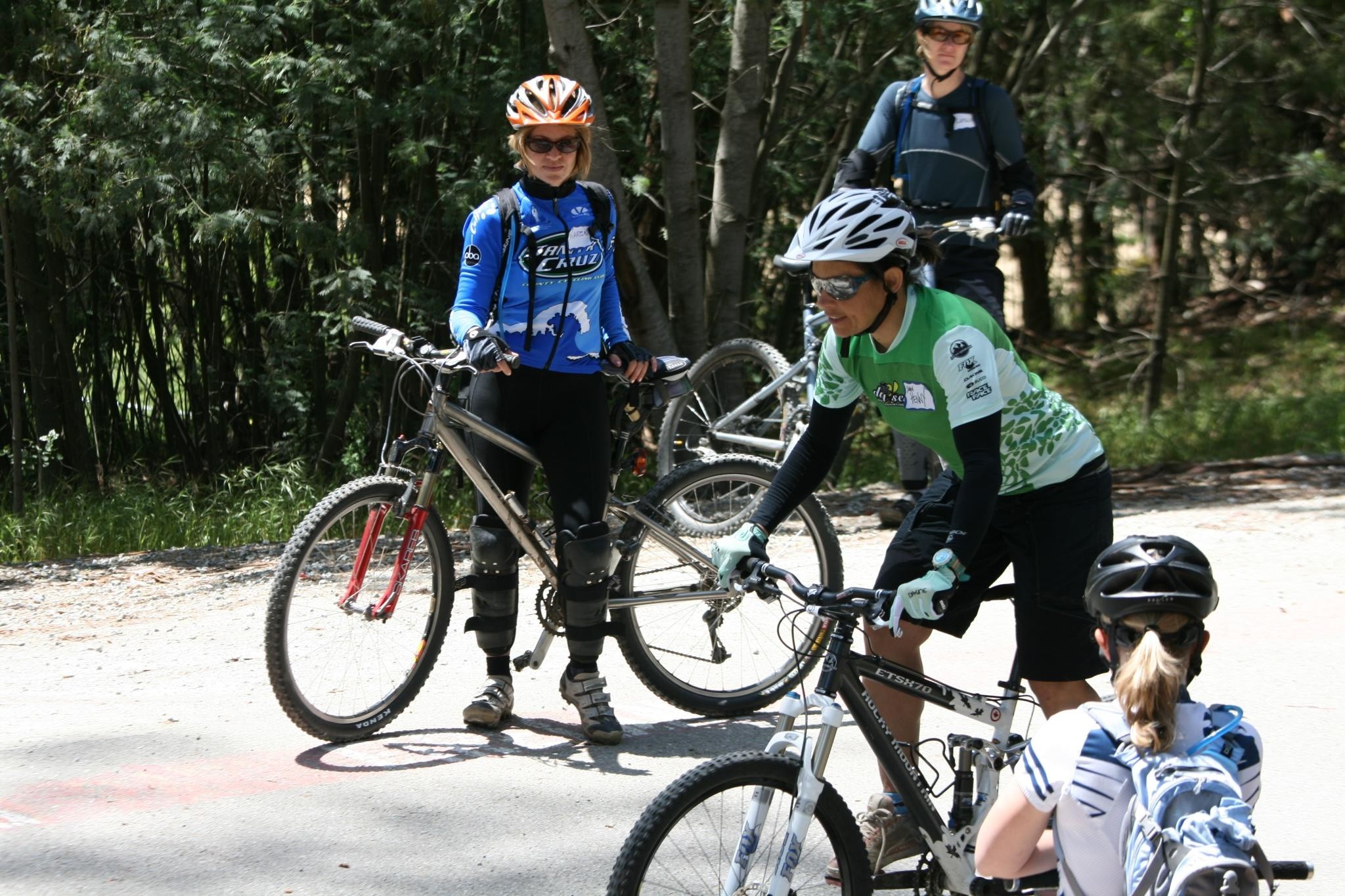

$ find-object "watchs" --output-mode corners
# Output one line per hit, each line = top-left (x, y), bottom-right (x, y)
(932, 548), (971, 582)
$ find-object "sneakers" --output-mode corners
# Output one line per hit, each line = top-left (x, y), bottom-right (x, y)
(461, 679), (514, 724)
(559, 665), (622, 744)
(824, 795), (934, 885)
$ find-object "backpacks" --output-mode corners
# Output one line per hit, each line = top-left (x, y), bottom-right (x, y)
(1077, 701), (1276, 896)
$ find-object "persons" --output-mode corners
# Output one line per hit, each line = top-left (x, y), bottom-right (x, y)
(974, 535), (1263, 896)
(448, 74), (657, 744)
(712, 187), (1113, 887)
(832, 0), (1039, 524)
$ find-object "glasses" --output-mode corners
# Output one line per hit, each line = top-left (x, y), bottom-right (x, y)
(922, 26), (972, 45)
(807, 273), (876, 300)
(524, 137), (580, 153)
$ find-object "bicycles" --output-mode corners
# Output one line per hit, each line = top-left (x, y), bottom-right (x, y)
(605, 558), (1060, 896)
(262, 314), (847, 745)
(654, 218), (1051, 540)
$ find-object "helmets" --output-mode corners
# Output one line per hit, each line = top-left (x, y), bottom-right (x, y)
(783, 189), (917, 263)
(1084, 535), (1220, 622)
(915, 1), (982, 31)
(506, 74), (595, 129)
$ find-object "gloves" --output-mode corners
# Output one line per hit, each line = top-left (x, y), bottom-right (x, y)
(888, 565), (961, 638)
(999, 205), (1036, 238)
(710, 522), (768, 593)
